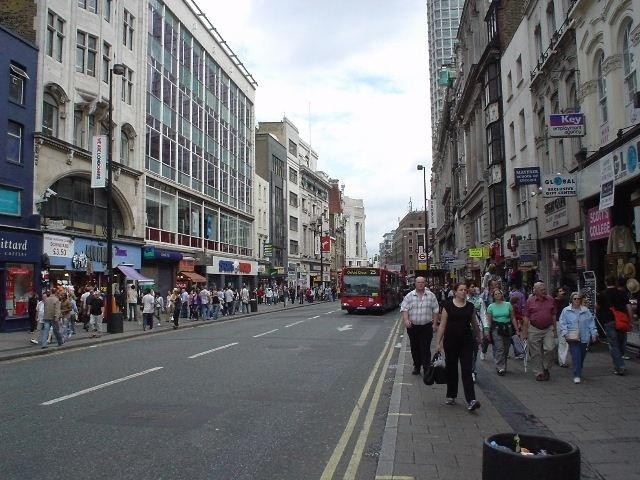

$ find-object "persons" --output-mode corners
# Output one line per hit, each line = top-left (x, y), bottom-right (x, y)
(400, 263), (632, 409)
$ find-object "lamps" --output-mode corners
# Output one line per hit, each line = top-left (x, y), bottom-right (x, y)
(574, 148), (597, 169)
(516, 172), (561, 206)
(617, 123), (640, 139)
(114, 167), (122, 181)
(634, 92), (640, 108)
(566, 233), (576, 252)
(66, 150), (75, 165)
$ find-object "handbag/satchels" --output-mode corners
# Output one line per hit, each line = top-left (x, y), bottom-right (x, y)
(614, 311), (631, 332)
(435, 357), (448, 385)
(565, 328), (580, 342)
(422, 360), (435, 386)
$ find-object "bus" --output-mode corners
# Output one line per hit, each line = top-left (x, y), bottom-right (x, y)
(339, 266), (404, 314)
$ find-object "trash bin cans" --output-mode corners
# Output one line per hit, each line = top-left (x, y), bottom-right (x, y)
(250, 299), (257, 312)
(308, 296), (314, 303)
(111, 312), (123, 333)
(482, 431), (581, 480)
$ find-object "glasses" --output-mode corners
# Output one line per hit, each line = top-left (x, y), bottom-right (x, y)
(573, 297), (581, 301)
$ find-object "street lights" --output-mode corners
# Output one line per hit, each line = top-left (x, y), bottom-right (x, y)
(257, 232), (267, 303)
(317, 212), (331, 282)
(416, 164), (429, 283)
(105, 64), (127, 324)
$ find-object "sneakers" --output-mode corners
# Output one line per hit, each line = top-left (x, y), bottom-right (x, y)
(28, 336), (53, 348)
(535, 370), (552, 382)
(575, 377), (581, 384)
(468, 399), (481, 411)
(472, 349), (511, 383)
(445, 396), (456, 405)
(411, 366), (421, 375)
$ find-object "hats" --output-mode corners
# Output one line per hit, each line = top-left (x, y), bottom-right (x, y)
(623, 262), (636, 279)
(626, 278), (640, 293)
